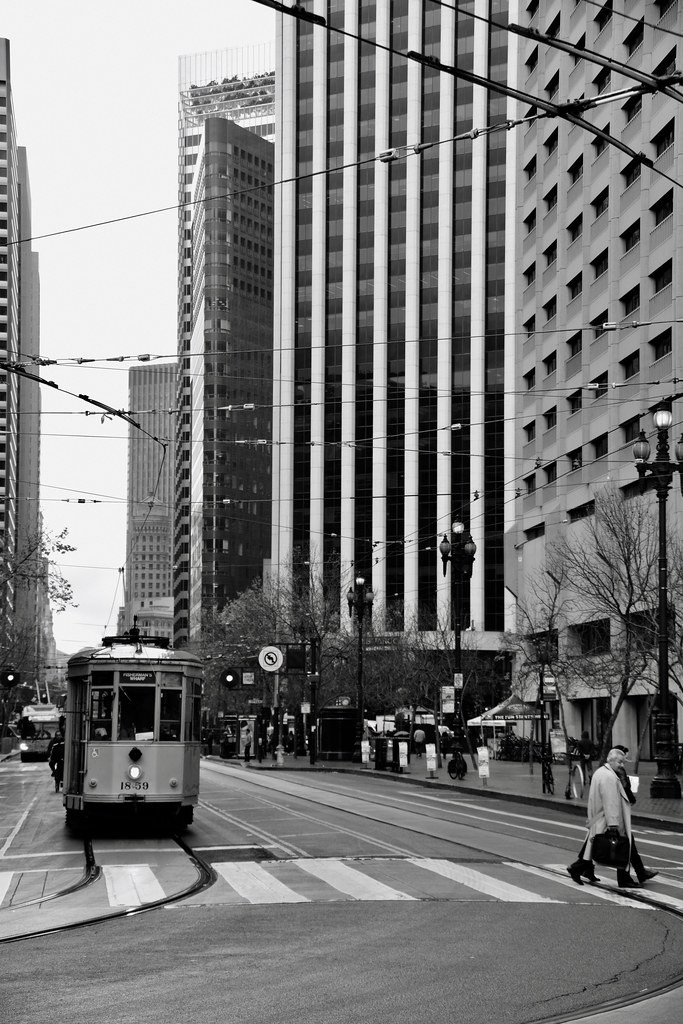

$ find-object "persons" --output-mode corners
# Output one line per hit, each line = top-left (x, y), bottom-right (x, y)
(441, 732), (449, 760)
(268, 731), (295, 760)
(566, 745), (659, 888)
(244, 729), (252, 762)
(48, 731), (64, 777)
(577, 731), (596, 786)
(413, 726), (425, 758)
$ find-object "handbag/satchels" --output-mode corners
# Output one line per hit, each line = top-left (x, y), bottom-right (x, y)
(591, 829), (630, 869)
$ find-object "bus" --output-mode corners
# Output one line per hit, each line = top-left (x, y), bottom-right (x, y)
(56, 616), (205, 830)
(18, 704), (59, 763)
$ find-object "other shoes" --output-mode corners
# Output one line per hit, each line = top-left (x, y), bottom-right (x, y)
(583, 869), (600, 882)
(637, 871), (659, 882)
(51, 770), (55, 776)
(567, 868), (585, 885)
(617, 877), (644, 888)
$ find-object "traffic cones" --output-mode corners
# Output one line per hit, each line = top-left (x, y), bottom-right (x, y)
(629, 395), (682, 799)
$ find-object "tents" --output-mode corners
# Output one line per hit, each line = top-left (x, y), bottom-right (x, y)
(467, 694), (549, 766)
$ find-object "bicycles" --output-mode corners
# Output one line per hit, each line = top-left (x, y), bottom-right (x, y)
(497, 732), (598, 766)
(448, 752), (467, 780)
(543, 755), (556, 795)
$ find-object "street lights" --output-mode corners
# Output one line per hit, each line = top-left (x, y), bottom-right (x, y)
(438, 521), (476, 758)
(345, 574), (373, 761)
